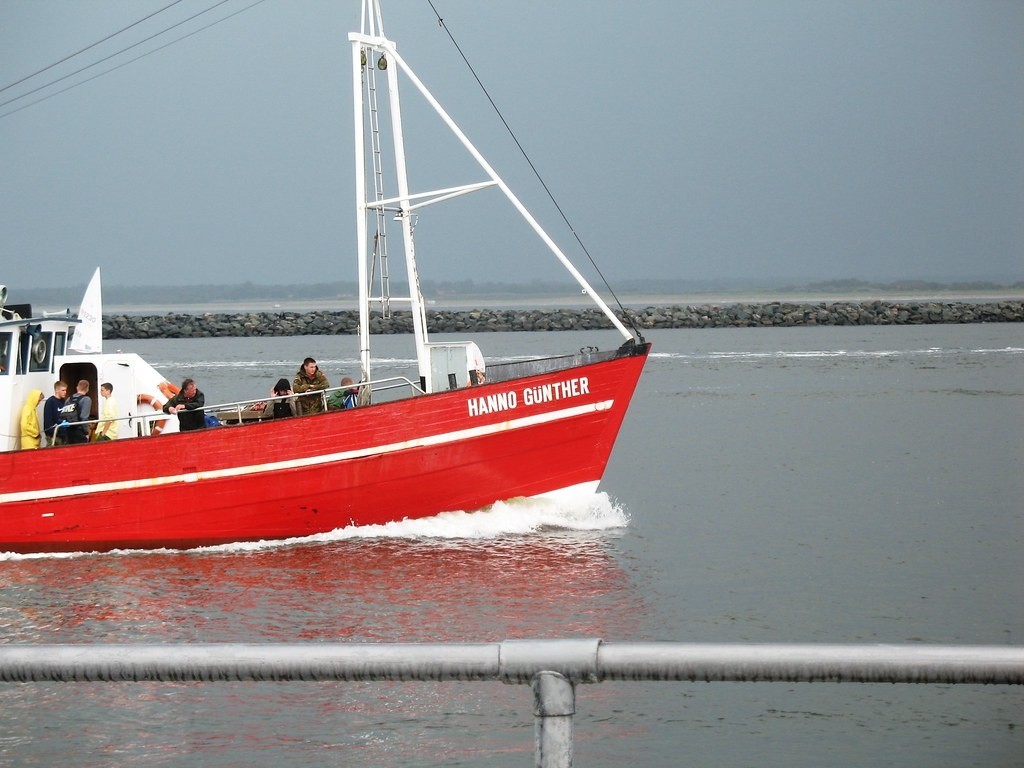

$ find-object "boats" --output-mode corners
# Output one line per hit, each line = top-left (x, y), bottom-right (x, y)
(0, 1), (655, 563)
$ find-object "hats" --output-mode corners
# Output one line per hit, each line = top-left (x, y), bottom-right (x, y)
(273, 378), (291, 391)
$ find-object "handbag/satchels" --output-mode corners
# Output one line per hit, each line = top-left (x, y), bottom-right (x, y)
(273, 403), (291, 417)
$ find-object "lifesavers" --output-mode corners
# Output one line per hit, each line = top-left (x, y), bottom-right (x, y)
(137, 393), (168, 438)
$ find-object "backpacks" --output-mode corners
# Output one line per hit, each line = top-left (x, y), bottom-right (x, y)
(59, 395), (87, 432)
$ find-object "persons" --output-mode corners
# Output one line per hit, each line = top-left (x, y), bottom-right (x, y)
(162, 379), (207, 432)
(264, 357), (359, 419)
(21, 380), (120, 449)
